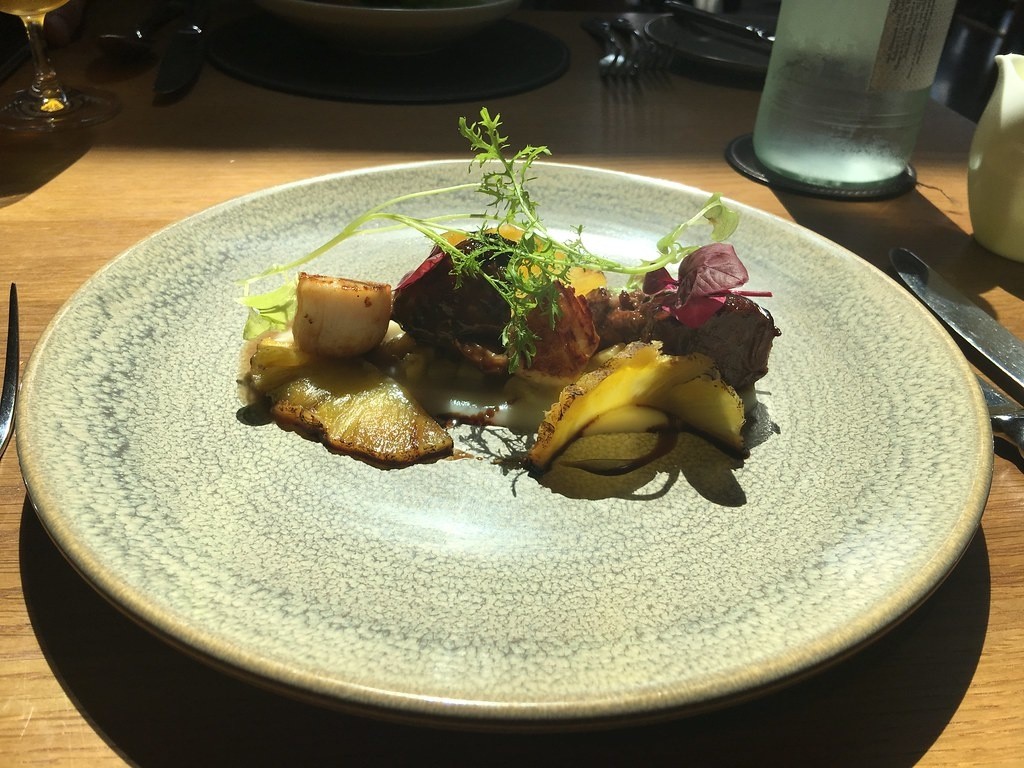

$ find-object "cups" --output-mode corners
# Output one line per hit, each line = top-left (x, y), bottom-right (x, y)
(966, 53), (1023, 262)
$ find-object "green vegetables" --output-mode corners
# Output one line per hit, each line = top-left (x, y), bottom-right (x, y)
(244, 107), (772, 376)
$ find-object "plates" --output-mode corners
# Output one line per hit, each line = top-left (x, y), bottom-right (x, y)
(644, 6), (777, 77)
(19, 156), (992, 717)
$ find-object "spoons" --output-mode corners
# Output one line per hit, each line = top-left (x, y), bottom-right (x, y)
(96, 0), (194, 60)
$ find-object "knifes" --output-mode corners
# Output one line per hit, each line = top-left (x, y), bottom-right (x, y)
(662, 0), (776, 44)
(889, 246), (1024, 387)
(971, 371), (1024, 460)
(152, 0), (210, 95)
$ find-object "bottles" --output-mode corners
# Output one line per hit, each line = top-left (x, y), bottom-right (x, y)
(751, 0), (958, 190)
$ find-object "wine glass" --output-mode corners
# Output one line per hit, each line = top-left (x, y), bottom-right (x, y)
(1, 0), (122, 133)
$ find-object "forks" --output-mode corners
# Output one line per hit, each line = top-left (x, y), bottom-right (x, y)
(0, 282), (19, 464)
(580, 17), (673, 80)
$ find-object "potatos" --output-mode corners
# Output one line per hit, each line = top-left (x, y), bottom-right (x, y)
(293, 272), (393, 357)
(245, 322), (744, 471)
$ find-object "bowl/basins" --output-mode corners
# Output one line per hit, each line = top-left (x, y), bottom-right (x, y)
(246, 0), (521, 56)
